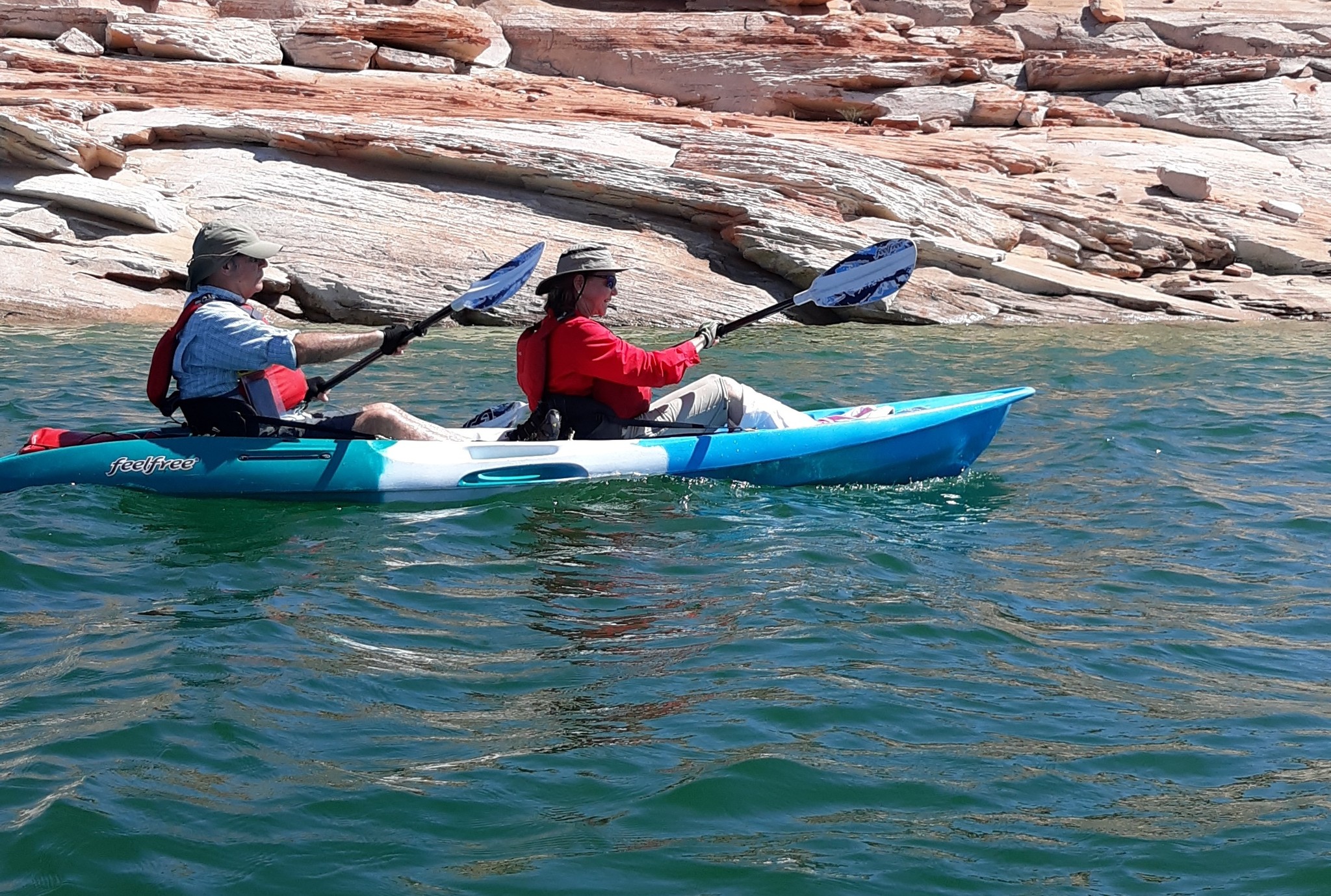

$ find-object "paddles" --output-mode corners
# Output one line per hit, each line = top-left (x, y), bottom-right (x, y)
(315, 240), (547, 394)
(460, 237), (919, 428)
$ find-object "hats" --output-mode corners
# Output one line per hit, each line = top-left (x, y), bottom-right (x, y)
(185, 221), (283, 291)
(535, 242), (630, 296)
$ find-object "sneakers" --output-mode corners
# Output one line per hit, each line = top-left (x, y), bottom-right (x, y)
(506, 402), (547, 442)
(523, 409), (560, 442)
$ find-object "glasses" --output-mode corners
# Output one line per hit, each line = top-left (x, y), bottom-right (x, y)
(249, 256), (261, 262)
(586, 274), (617, 289)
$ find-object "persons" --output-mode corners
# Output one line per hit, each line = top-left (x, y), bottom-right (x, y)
(517, 244), (893, 439)
(172, 220), (563, 442)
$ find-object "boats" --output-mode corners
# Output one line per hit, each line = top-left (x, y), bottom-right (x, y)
(1, 386), (1037, 503)
